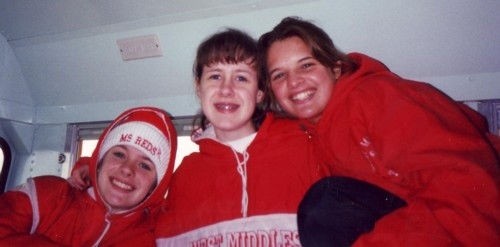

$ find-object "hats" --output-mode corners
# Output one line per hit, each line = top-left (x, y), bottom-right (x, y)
(99, 111), (170, 184)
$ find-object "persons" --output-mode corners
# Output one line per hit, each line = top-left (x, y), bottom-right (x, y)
(70, 26), (325, 247)
(0, 106), (177, 247)
(257, 16), (500, 247)
